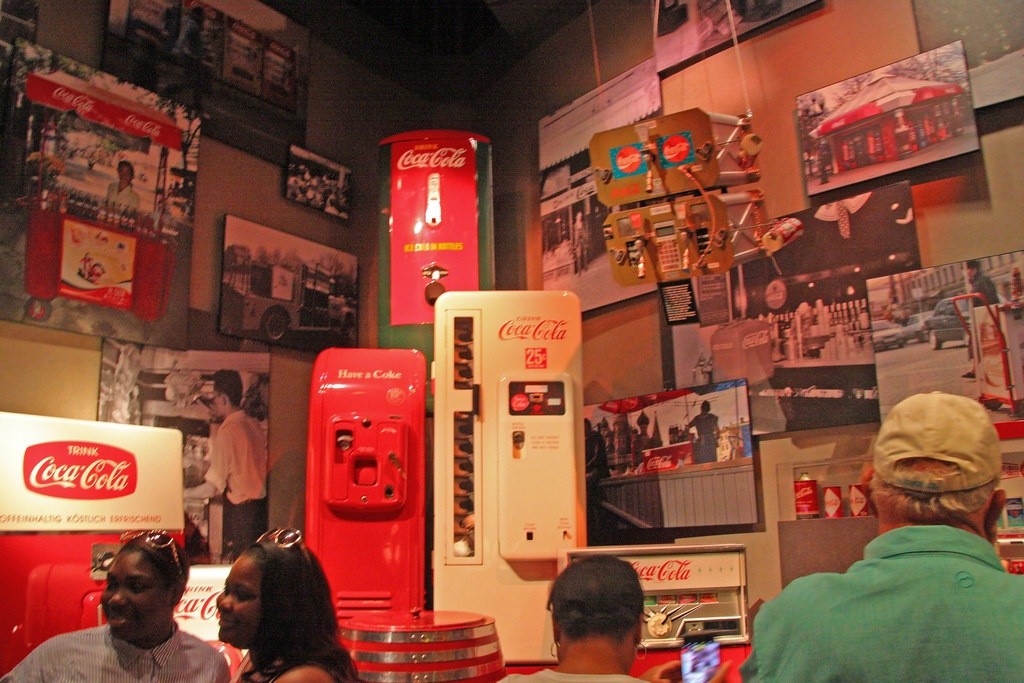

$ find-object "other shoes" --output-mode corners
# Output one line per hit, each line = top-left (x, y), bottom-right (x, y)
(962, 372), (976, 378)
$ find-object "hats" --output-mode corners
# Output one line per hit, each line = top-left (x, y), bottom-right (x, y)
(967, 260), (980, 268)
(873, 391), (1002, 496)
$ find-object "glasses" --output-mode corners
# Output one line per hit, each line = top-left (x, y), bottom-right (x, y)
(209, 393), (223, 404)
(120, 528), (187, 584)
(257, 527), (314, 573)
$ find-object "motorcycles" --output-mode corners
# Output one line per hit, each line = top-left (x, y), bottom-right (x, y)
(87, 152), (97, 170)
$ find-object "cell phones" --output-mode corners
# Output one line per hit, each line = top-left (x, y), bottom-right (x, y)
(680, 640), (719, 683)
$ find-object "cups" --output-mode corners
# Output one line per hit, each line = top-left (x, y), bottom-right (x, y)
(822, 486), (844, 518)
(793, 480), (821, 519)
(849, 485), (868, 516)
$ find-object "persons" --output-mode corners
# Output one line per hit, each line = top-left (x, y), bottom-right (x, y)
(215, 527), (356, 683)
(962, 261), (1000, 379)
(0, 532), (230, 683)
(289, 168), (350, 219)
(684, 399), (720, 463)
(163, 6), (211, 121)
(184, 370), (268, 564)
(106, 160), (139, 219)
(740, 391), (1024, 683)
(571, 212), (591, 276)
(496, 555), (652, 683)
(584, 417), (610, 488)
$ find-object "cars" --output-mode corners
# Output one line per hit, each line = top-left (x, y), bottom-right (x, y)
(925, 294), (1009, 350)
(895, 311), (935, 347)
(222, 243), (355, 342)
(113, 149), (179, 191)
(871, 319), (906, 351)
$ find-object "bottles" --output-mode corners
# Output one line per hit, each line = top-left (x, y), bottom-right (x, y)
(798, 470), (810, 480)
(667, 422), (690, 446)
(43, 178), (157, 241)
(758, 299), (870, 361)
